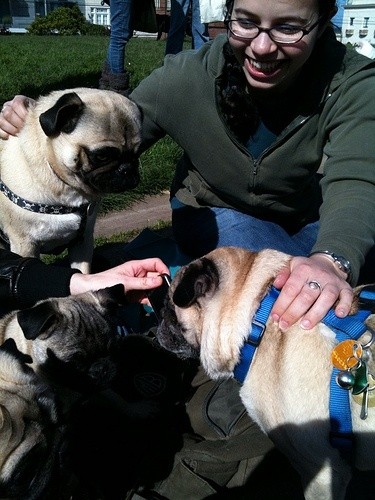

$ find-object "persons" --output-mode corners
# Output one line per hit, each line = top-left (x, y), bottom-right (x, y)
(0, 0), (375, 331)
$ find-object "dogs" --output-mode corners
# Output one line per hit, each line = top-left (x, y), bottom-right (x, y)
(0, 88), (143, 275)
(157, 246), (375, 500)
(0, 283), (126, 500)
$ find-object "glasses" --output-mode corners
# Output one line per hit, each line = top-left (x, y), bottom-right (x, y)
(224, 0), (330, 45)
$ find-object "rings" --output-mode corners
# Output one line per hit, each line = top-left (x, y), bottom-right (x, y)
(305, 281), (323, 295)
(1, 104), (10, 113)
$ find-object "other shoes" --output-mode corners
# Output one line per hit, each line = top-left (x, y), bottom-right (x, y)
(98, 63), (133, 97)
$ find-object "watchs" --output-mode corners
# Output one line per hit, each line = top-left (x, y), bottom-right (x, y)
(308, 250), (352, 282)
